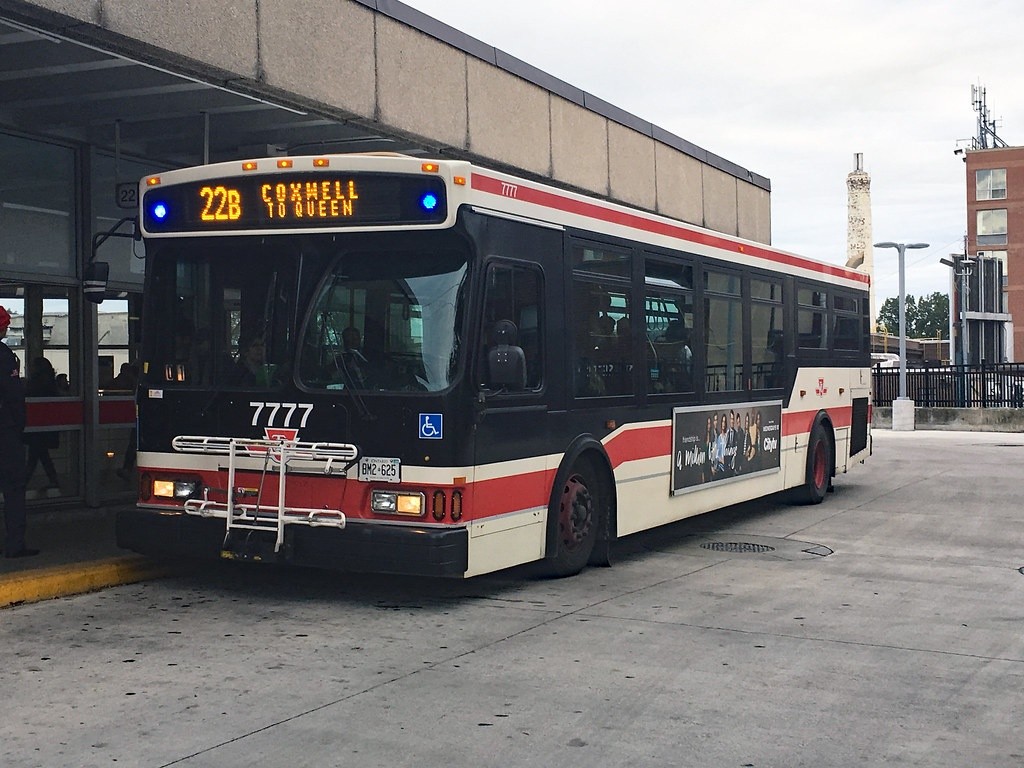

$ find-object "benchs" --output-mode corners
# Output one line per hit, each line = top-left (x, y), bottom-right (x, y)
(580, 330), (690, 394)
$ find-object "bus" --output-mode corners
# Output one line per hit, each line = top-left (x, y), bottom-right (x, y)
(113, 150), (874, 586)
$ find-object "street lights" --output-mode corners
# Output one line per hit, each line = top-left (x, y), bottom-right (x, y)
(873, 241), (930, 433)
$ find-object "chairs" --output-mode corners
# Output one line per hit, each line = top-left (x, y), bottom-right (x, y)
(486, 320), (527, 390)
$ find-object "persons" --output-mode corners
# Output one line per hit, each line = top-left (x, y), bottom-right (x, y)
(705, 411), (764, 480)
(22, 327), (377, 490)
(1, 307), (38, 559)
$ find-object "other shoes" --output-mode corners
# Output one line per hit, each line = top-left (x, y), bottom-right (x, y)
(47, 483), (61, 489)
(6, 549), (40, 558)
(117, 467), (131, 480)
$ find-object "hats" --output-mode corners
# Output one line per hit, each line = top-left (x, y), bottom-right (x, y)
(0, 305), (11, 330)
